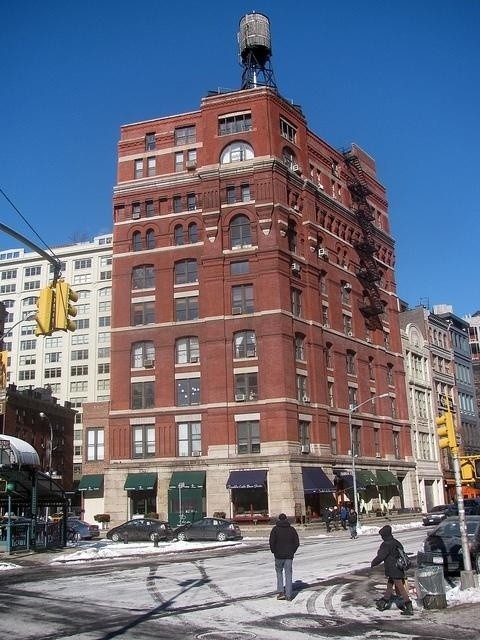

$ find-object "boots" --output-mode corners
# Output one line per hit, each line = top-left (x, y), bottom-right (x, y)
(376, 597), (387, 611)
(401, 600), (414, 615)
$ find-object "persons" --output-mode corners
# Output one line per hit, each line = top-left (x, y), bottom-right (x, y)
(269, 512), (300, 601)
(370, 525), (414, 616)
(323, 504), (359, 540)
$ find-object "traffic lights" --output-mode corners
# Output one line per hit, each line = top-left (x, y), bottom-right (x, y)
(434, 413), (457, 449)
(34, 282), (78, 337)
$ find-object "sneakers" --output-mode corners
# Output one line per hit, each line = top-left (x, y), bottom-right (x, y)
(277, 592), (295, 601)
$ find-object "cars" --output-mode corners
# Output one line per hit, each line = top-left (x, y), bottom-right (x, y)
(454, 499), (480, 515)
(422, 504), (456, 526)
(57, 519), (100, 541)
(106, 518), (173, 542)
(174, 517), (243, 541)
(417, 515), (480, 578)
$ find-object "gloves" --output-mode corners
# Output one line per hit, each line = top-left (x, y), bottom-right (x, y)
(371, 562), (377, 569)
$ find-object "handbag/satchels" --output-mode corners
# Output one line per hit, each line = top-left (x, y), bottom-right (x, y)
(395, 548), (411, 571)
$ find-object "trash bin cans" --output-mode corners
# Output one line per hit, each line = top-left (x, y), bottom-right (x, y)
(213, 512), (226, 519)
(415, 566), (447, 610)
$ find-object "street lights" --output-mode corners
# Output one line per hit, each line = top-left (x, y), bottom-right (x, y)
(39, 412), (54, 477)
(348, 393), (389, 531)
